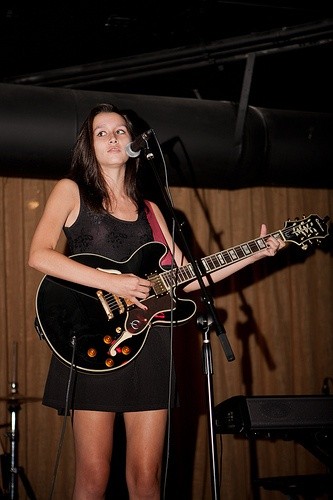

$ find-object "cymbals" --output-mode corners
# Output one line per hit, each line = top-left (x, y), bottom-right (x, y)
(0, 393), (42, 403)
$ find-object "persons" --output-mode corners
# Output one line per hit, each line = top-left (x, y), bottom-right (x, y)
(26, 102), (290, 500)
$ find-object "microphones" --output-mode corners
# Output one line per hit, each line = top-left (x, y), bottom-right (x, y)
(124, 128), (153, 157)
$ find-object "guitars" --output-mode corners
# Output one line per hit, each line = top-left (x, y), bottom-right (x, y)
(35, 212), (333, 376)
